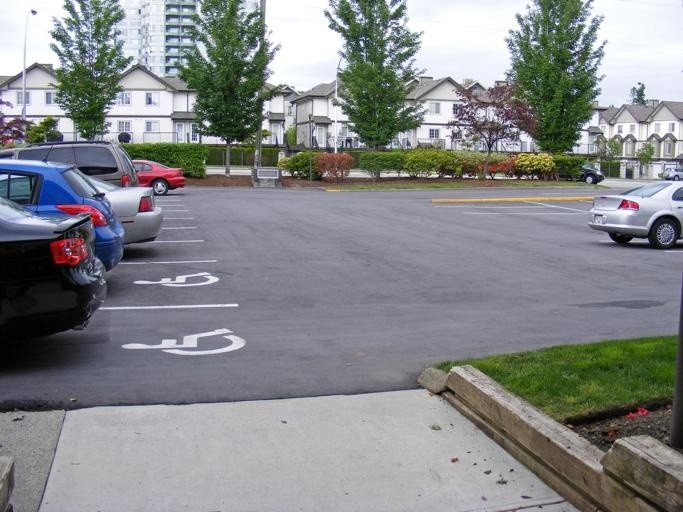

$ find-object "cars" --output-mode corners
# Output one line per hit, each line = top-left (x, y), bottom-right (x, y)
(662, 167), (683, 182)
(556, 164), (605, 186)
(0, 170), (165, 245)
(0, 160), (125, 273)
(130, 158), (186, 200)
(0, 197), (109, 345)
(581, 179), (683, 248)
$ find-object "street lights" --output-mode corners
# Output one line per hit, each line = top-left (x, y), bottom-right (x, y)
(333, 44), (356, 153)
(22, 8), (39, 147)
(308, 114), (314, 183)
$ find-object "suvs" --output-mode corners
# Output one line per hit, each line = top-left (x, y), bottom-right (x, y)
(0, 140), (141, 191)
